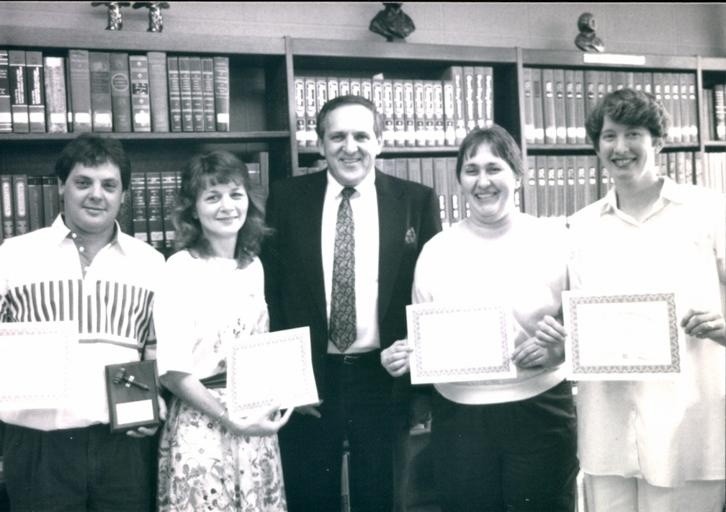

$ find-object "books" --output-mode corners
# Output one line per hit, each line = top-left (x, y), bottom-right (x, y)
(0, 48), (229, 132)
(295, 65), (494, 147)
(0, 152), (269, 249)
(522, 67), (726, 145)
(300, 152), (726, 226)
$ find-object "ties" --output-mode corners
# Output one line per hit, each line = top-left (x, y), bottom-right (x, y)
(329, 187), (358, 353)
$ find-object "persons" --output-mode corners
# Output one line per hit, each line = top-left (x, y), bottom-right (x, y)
(532, 89), (725, 510)
(149, 147), (298, 511)
(259, 91), (442, 511)
(0, 127), (170, 512)
(379, 122), (576, 511)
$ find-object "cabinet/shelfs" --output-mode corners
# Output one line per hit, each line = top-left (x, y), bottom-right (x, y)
(0, 25), (726, 260)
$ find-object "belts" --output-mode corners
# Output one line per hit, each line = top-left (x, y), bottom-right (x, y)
(327, 348), (381, 367)
